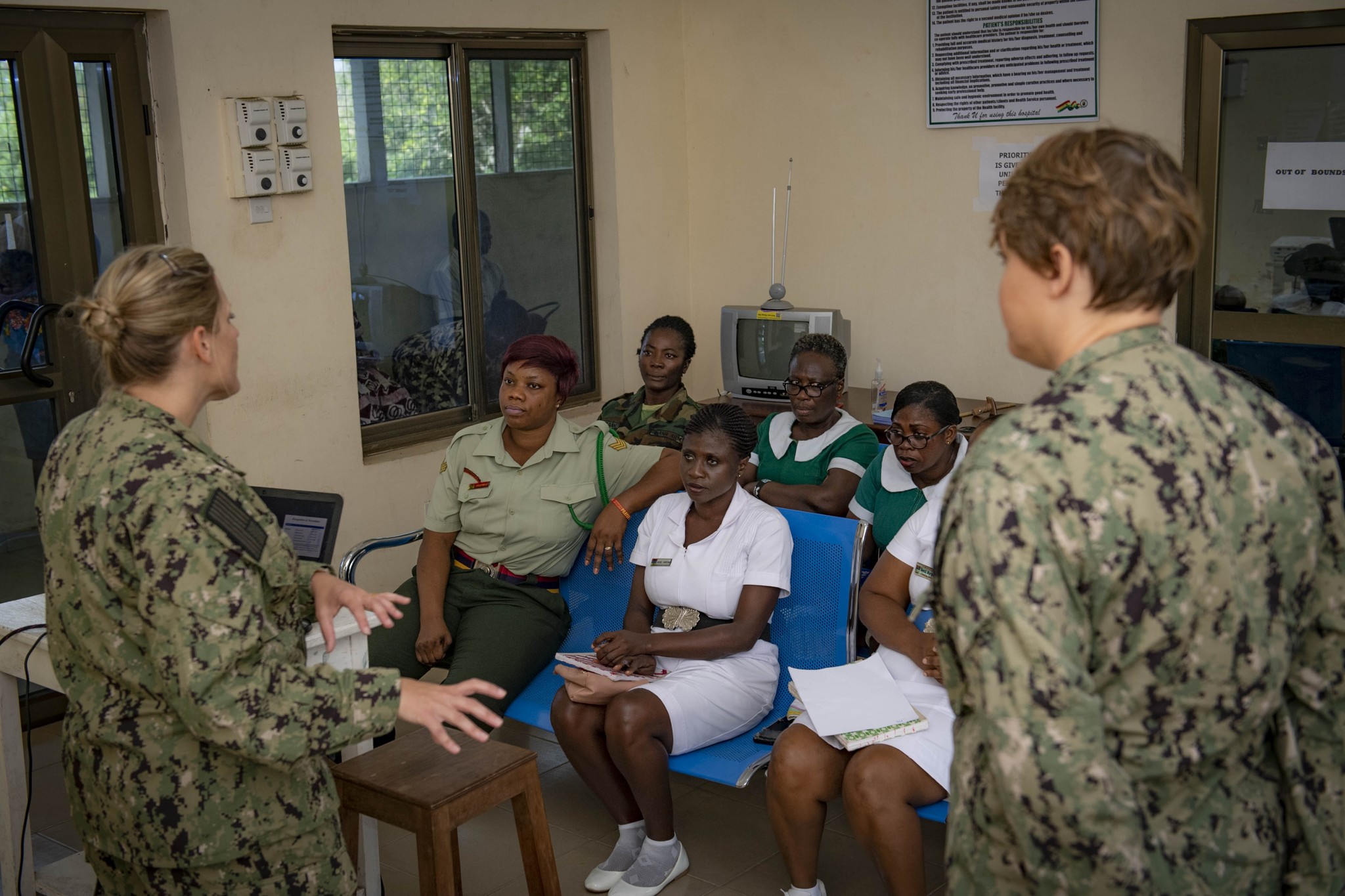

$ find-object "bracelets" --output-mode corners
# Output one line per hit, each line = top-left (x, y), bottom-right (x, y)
(753, 478), (770, 498)
(611, 499), (631, 520)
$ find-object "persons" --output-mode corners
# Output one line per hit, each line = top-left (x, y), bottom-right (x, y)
(365, 335), (685, 748)
(932, 124), (1345, 896)
(548, 400), (794, 896)
(739, 332), (878, 524)
(766, 405), (1023, 895)
(844, 382), (970, 654)
(597, 315), (705, 451)
(428, 208), (501, 326)
(32, 245), (506, 895)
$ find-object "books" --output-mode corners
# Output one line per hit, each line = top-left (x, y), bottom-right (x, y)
(555, 649), (671, 681)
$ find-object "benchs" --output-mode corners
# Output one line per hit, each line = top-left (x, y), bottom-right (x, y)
(1211, 342), (1345, 459)
(336, 507), (948, 824)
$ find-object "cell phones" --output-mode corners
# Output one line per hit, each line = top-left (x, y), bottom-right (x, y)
(753, 716), (795, 747)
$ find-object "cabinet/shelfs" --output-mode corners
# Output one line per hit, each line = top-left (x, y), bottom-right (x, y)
(0, 592), (381, 896)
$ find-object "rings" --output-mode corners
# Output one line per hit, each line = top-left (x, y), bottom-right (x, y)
(604, 546), (613, 550)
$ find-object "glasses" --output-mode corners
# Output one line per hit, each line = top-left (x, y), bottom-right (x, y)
(883, 424), (951, 449)
(782, 376), (839, 398)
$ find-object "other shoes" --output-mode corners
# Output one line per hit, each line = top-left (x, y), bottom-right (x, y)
(607, 840), (690, 896)
(584, 862), (627, 893)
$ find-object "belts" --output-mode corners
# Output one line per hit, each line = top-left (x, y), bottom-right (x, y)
(452, 545), (560, 593)
(653, 605), (771, 643)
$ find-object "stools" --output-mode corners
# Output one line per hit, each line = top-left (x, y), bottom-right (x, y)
(329, 725), (561, 896)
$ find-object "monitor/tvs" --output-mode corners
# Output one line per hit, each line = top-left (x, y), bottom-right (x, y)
(249, 485), (343, 563)
(721, 158), (853, 403)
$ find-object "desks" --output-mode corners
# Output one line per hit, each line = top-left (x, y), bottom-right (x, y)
(700, 388), (1030, 447)
